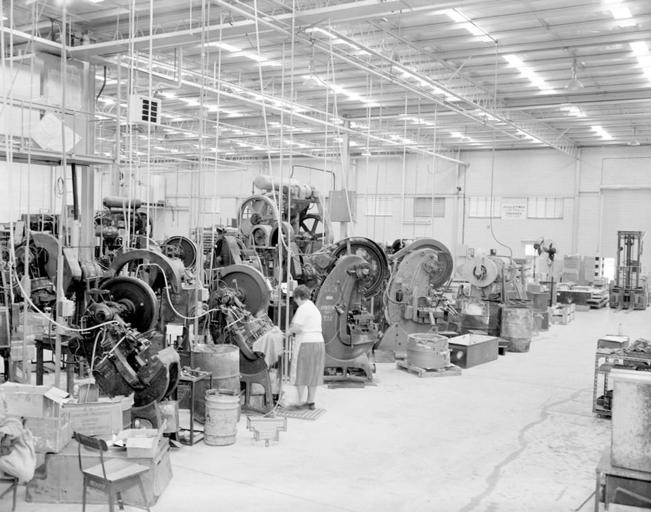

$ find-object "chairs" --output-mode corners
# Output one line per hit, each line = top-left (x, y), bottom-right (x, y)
(72, 431), (152, 511)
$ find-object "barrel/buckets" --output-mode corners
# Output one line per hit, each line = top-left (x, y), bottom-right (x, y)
(203, 388), (239, 447)
(608, 366), (651, 474)
(194, 343), (241, 424)
(501, 307), (532, 353)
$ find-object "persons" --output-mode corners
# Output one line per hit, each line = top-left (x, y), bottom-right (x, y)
(286, 283), (326, 411)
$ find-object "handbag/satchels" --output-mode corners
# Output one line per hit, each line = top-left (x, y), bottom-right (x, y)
(0, 418), (36, 481)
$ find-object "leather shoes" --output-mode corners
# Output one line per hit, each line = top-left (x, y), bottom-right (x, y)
(304, 402), (314, 410)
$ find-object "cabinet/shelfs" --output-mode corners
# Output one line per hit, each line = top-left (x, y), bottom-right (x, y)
(594, 353), (651, 417)
